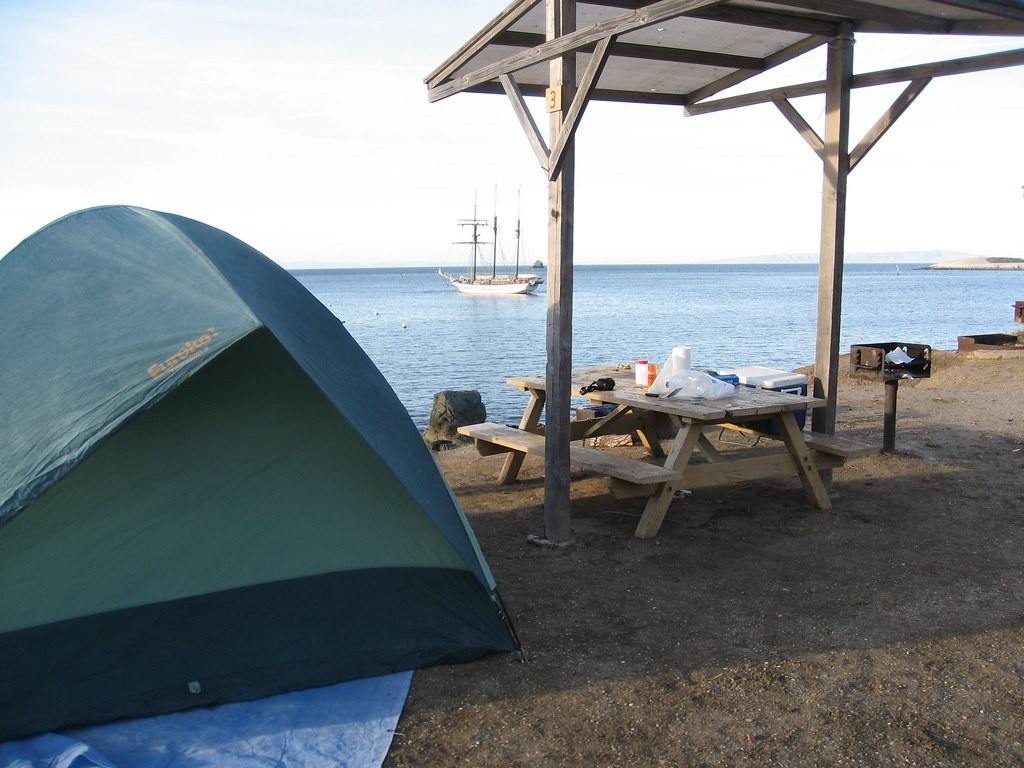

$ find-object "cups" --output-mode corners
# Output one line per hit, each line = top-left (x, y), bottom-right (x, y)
(634, 360), (648, 384)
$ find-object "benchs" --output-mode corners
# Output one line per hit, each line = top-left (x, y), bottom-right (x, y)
(457, 421), (880, 497)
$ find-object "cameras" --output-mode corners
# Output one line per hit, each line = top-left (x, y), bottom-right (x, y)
(597, 377), (615, 391)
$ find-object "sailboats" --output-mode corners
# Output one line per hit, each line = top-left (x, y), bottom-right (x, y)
(435, 185), (545, 294)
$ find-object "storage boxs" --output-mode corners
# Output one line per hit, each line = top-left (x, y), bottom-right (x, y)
(720, 366), (807, 435)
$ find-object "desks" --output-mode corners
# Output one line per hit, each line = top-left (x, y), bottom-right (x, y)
(498, 363), (832, 537)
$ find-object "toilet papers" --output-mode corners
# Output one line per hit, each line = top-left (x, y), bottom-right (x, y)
(647, 343), (690, 397)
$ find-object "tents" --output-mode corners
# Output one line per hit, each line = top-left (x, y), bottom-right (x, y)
(0, 205), (525, 743)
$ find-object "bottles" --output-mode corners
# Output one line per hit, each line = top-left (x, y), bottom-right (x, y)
(648, 363), (656, 389)
